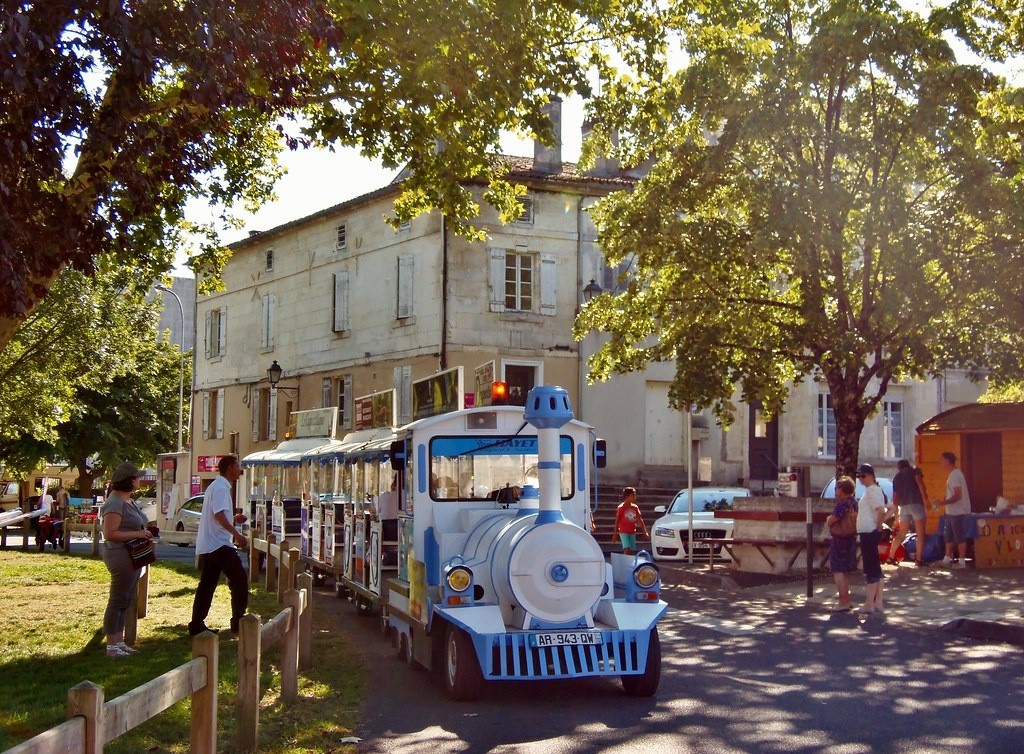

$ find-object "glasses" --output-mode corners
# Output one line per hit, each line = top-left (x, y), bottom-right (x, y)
(858, 473), (872, 478)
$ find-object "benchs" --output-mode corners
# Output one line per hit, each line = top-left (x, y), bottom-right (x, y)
(253, 506), (398, 571)
(694, 536), (863, 572)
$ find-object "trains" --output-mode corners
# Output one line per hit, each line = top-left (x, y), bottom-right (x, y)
(239, 380), (671, 706)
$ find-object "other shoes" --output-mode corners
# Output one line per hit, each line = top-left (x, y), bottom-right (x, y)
(826, 603), (853, 612)
(853, 604), (883, 614)
(105, 645), (129, 656)
(189, 628), (219, 637)
(119, 644), (139, 652)
(936, 561), (953, 568)
(950, 564), (966, 569)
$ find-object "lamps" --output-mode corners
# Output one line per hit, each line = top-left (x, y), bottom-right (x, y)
(265, 360), (301, 411)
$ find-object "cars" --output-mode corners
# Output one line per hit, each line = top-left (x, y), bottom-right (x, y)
(650, 485), (754, 562)
(168, 492), (205, 548)
(817, 475), (893, 503)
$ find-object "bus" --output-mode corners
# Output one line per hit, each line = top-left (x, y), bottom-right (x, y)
(0, 465), (81, 526)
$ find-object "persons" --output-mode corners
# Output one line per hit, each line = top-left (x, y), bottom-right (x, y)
(931, 452), (972, 569)
(377, 473), (399, 521)
(36, 485), (71, 525)
(853, 463), (896, 613)
(188, 455), (262, 634)
(825, 475), (858, 611)
(885, 460), (932, 567)
(101, 463), (160, 658)
(611, 486), (649, 555)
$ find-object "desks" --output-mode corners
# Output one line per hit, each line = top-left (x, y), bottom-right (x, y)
(939, 513), (1024, 569)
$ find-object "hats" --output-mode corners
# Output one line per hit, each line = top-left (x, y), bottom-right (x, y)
(112, 463), (146, 483)
(856, 463), (873, 474)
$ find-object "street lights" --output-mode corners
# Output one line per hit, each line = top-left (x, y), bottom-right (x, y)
(154, 283), (185, 453)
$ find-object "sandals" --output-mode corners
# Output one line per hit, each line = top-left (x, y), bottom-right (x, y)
(886, 557), (897, 566)
(915, 559), (928, 567)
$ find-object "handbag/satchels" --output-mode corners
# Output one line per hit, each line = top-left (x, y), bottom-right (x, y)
(126, 536), (157, 570)
(831, 498), (859, 537)
